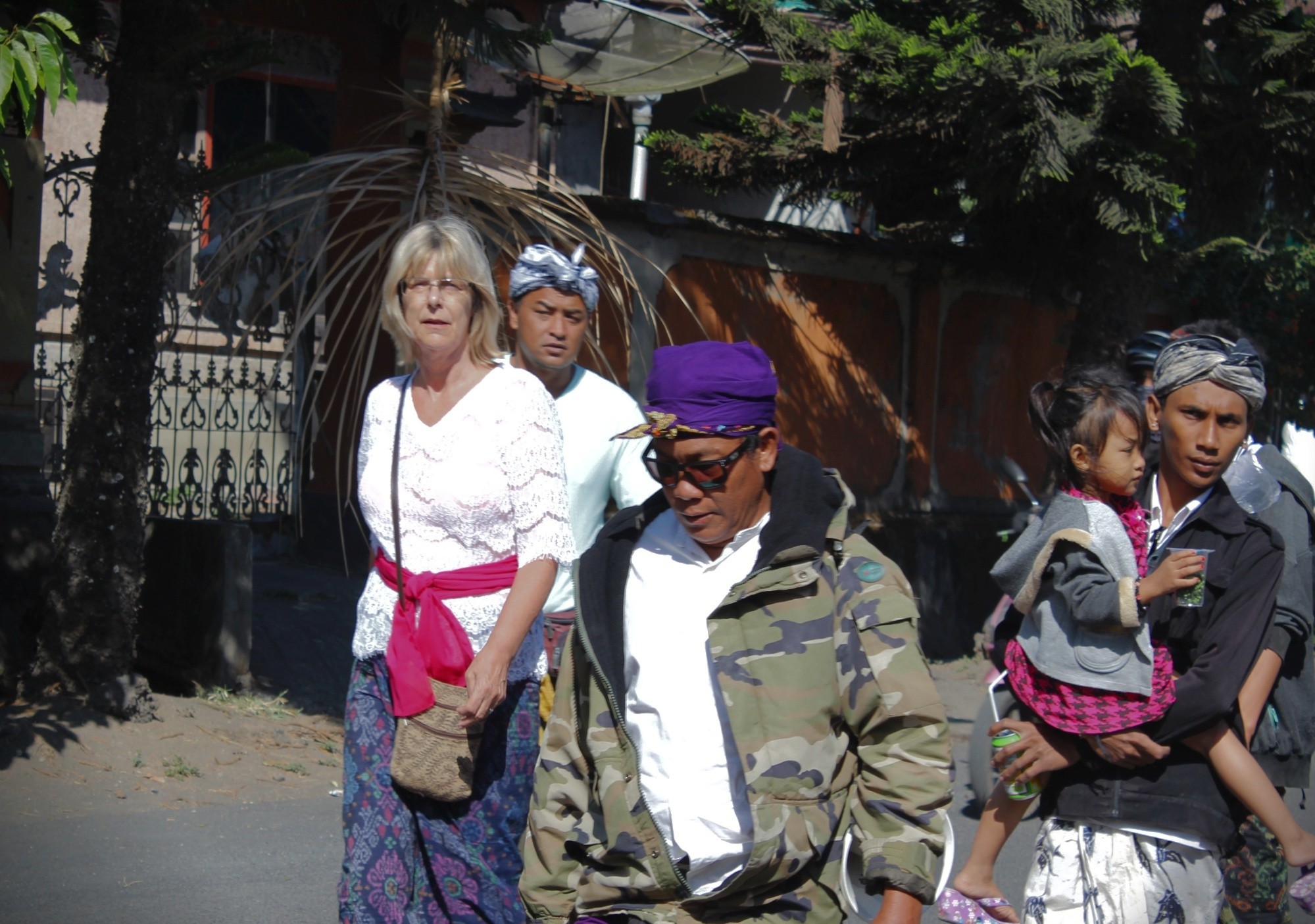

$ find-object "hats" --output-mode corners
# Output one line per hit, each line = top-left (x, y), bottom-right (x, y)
(609, 342), (776, 441)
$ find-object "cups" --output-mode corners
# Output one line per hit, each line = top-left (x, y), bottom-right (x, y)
(1166, 547), (1216, 608)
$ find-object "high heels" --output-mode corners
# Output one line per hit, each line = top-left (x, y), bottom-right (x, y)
(1289, 860), (1315, 916)
(937, 887), (1019, 924)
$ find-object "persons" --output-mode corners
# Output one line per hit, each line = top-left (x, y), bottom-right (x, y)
(491, 241), (663, 750)
(338, 214), (579, 924)
(937, 319), (1315, 924)
(518, 341), (956, 924)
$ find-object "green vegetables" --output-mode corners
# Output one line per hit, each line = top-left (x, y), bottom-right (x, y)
(1175, 573), (1205, 603)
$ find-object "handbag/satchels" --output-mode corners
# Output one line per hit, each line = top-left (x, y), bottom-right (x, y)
(543, 611), (577, 677)
(389, 676), (486, 802)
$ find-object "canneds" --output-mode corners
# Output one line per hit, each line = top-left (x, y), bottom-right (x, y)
(992, 730), (1044, 801)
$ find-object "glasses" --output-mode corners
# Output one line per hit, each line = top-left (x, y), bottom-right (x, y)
(398, 278), (474, 297)
(642, 437), (752, 489)
